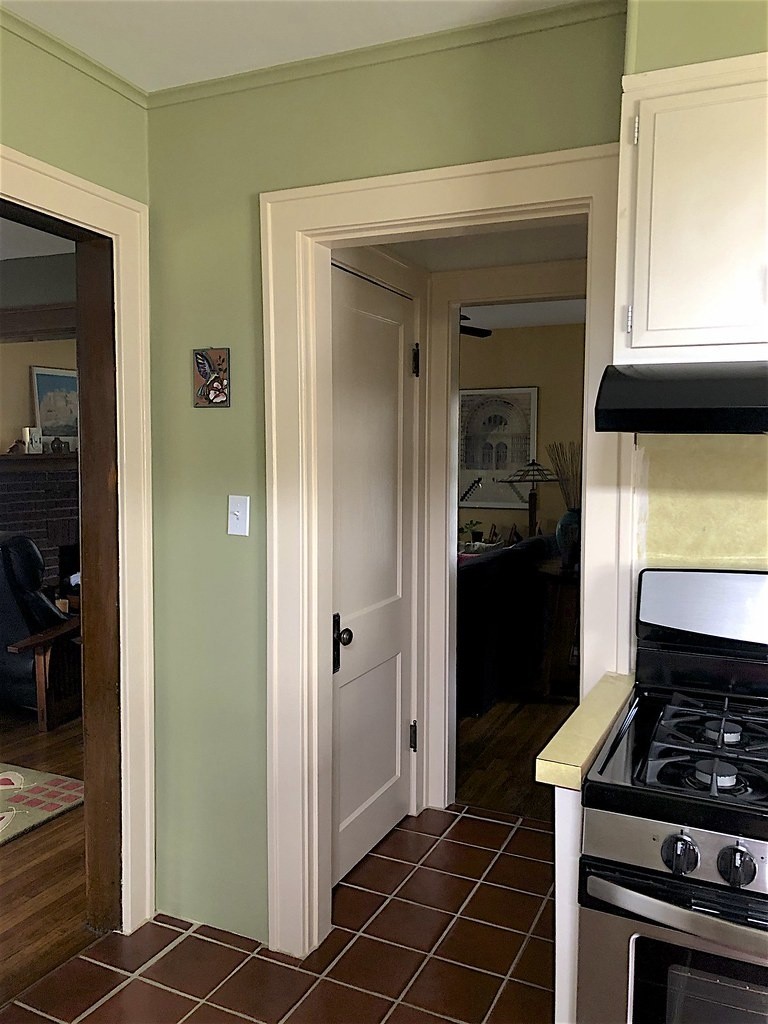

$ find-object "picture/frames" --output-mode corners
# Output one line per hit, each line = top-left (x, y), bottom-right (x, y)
(190, 346), (231, 409)
(28, 364), (80, 450)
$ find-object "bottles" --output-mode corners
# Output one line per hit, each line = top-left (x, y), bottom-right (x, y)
(51, 437), (63, 453)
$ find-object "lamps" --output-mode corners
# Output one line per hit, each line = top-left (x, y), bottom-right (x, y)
(495, 456), (564, 538)
(460, 383), (541, 513)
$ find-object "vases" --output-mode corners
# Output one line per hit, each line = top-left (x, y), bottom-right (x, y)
(555, 507), (580, 573)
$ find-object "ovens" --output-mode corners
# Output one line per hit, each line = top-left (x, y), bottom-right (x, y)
(574, 855), (768, 1024)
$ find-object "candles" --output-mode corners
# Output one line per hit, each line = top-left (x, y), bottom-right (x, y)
(54, 597), (70, 614)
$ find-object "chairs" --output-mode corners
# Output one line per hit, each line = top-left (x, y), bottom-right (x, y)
(0, 529), (87, 737)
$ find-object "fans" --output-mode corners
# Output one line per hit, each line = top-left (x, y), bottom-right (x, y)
(458, 313), (495, 341)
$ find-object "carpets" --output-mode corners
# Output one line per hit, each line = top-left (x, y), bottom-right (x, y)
(0, 760), (86, 849)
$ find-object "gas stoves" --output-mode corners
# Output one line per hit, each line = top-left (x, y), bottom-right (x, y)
(577, 564), (768, 894)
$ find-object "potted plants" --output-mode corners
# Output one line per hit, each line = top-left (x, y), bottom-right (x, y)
(463, 515), (485, 544)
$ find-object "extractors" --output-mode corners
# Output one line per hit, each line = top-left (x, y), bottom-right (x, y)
(594, 360), (768, 435)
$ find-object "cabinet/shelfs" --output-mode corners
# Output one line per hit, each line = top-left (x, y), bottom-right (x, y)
(610, 50), (768, 368)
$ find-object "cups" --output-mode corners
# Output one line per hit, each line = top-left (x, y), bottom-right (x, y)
(62, 441), (70, 453)
(55, 599), (68, 613)
